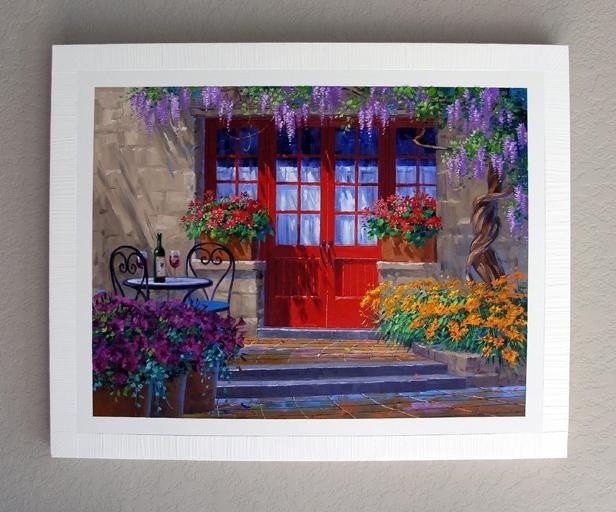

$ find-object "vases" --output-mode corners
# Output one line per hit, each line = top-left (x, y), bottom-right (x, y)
(199, 231), (259, 261)
(152, 372), (186, 416)
(380, 233), (439, 262)
(185, 356), (218, 413)
(92, 380), (152, 416)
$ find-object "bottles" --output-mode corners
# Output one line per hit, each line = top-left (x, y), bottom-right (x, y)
(151, 232), (167, 281)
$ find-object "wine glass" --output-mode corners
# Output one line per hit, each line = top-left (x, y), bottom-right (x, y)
(168, 249), (182, 281)
(135, 251), (148, 282)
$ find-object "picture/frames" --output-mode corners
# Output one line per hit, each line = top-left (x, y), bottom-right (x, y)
(49, 35), (569, 464)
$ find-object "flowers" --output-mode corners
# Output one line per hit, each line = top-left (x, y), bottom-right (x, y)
(91, 291), (170, 393)
(178, 189), (274, 245)
(200, 308), (249, 369)
(153, 298), (204, 374)
(362, 190), (444, 250)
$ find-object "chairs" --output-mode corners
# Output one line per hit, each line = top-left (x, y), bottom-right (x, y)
(110, 244), (151, 303)
(185, 241), (234, 317)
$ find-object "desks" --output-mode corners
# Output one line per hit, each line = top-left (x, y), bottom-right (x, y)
(122, 275), (213, 305)
